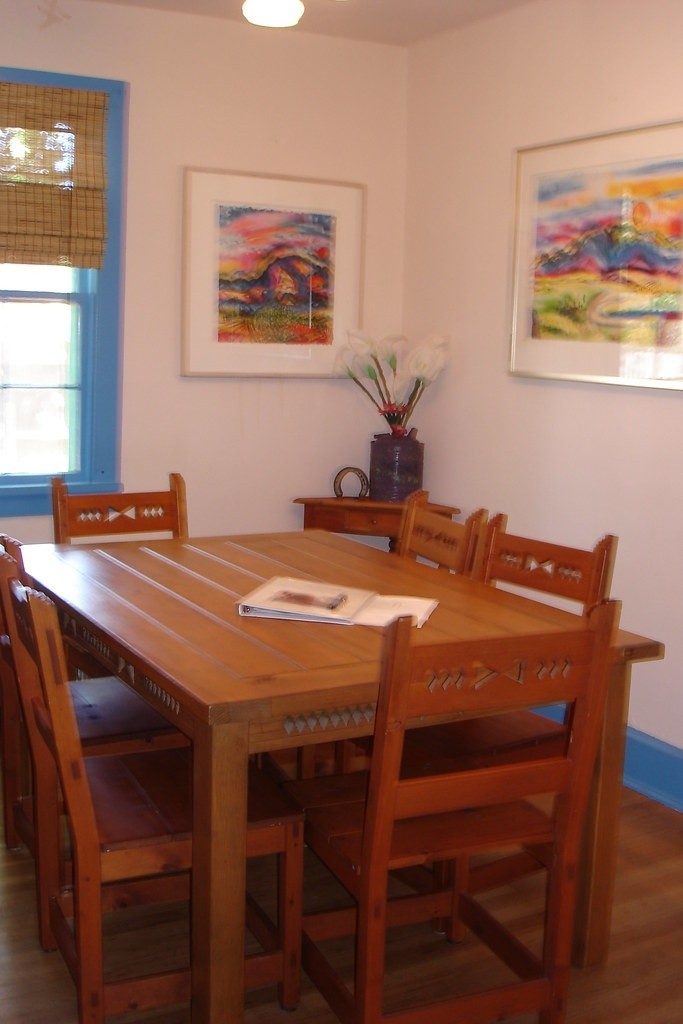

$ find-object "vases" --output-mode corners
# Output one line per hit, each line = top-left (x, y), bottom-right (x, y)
(369, 434), (424, 502)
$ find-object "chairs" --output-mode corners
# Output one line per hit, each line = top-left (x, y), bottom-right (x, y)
(0, 473), (621, 1024)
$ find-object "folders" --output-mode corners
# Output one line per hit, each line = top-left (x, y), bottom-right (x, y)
(232, 574), (377, 626)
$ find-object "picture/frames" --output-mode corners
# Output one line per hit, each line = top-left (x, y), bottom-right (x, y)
(509, 120), (683, 392)
(180, 166), (368, 380)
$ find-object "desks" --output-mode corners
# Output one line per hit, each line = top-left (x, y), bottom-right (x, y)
(294, 497), (461, 573)
(20, 528), (665, 1024)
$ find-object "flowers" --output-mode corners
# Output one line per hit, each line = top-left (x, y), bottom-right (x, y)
(333, 327), (446, 438)
